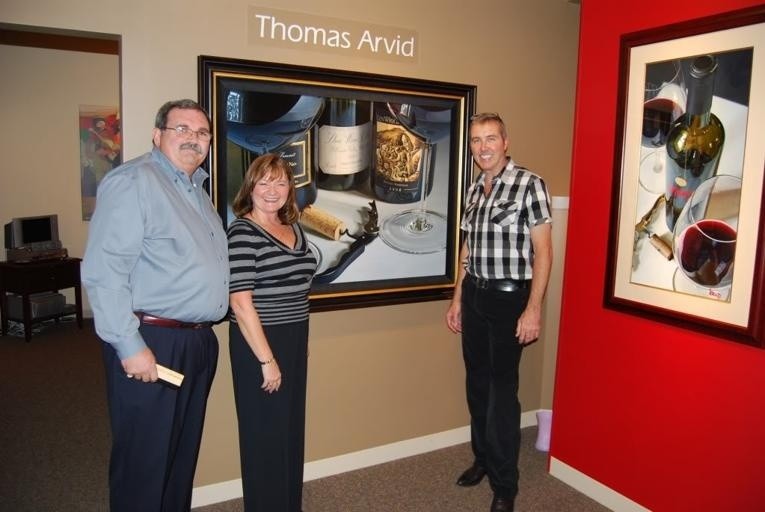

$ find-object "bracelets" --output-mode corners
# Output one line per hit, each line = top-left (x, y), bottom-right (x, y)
(257, 354), (277, 367)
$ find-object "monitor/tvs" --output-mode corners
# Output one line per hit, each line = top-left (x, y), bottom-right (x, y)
(4, 214), (59, 250)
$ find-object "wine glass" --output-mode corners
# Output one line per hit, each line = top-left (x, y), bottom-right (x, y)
(673, 175), (743, 301)
(639, 59), (686, 193)
(223, 89), (323, 277)
(378, 95), (452, 255)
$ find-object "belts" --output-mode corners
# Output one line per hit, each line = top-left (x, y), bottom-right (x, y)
(465, 272), (528, 292)
(133, 311), (214, 330)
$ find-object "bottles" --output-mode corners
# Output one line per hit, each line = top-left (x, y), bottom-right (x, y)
(241, 109), (318, 214)
(369, 101), (435, 205)
(665, 55), (723, 231)
(313, 94), (374, 191)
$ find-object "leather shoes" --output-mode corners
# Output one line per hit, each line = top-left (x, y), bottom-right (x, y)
(456, 461), (486, 487)
(490, 491), (516, 512)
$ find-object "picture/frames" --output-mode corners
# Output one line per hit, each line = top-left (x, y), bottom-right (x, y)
(601, 2), (765, 349)
(196, 50), (479, 316)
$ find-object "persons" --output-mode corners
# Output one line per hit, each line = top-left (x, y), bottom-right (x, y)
(223, 151), (323, 512)
(441, 111), (556, 511)
(79, 97), (232, 511)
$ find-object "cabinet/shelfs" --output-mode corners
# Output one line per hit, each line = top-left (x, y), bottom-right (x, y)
(0, 257), (86, 343)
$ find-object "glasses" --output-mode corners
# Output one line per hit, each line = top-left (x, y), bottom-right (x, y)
(160, 124), (213, 142)
